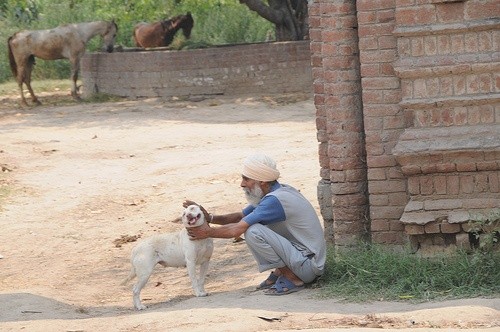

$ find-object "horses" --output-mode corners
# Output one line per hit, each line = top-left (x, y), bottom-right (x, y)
(7, 19), (118, 108)
(130, 11), (193, 48)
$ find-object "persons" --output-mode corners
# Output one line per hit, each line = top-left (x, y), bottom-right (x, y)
(182, 153), (329, 296)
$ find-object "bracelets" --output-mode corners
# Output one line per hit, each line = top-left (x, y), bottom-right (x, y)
(208, 212), (214, 225)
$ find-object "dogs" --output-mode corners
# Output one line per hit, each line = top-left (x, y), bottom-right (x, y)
(119, 205), (214, 311)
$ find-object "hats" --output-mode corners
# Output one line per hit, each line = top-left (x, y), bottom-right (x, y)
(242, 153), (279, 181)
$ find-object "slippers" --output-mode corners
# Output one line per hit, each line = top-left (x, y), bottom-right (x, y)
(258, 269), (282, 290)
(267, 273), (306, 295)
